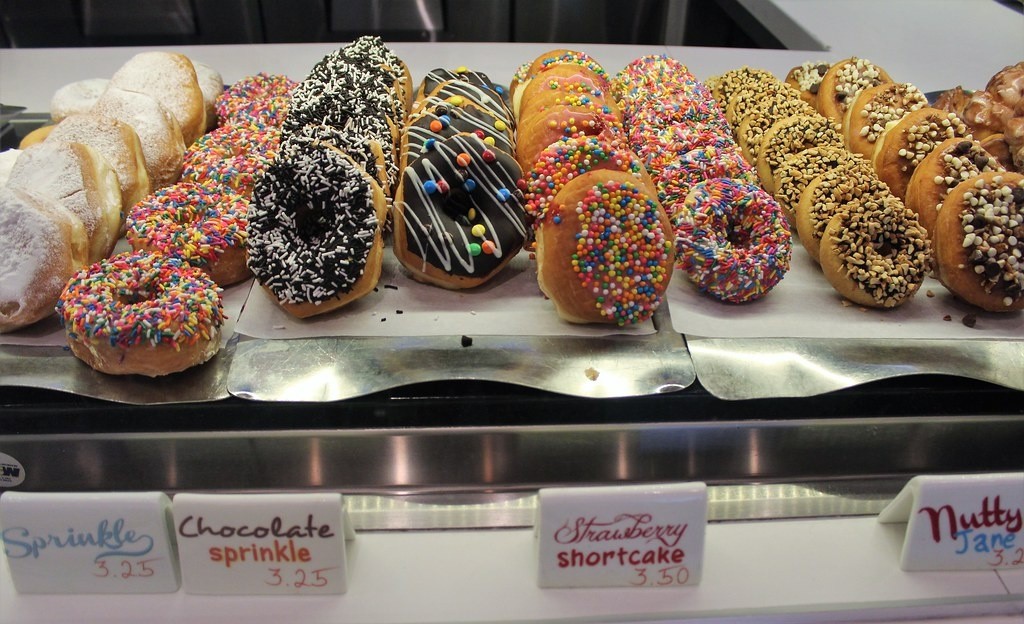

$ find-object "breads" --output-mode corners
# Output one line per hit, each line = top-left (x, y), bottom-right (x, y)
(0, 51), (221, 330)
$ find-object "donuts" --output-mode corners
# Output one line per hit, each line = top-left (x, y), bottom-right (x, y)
(54, 35), (1024, 376)
